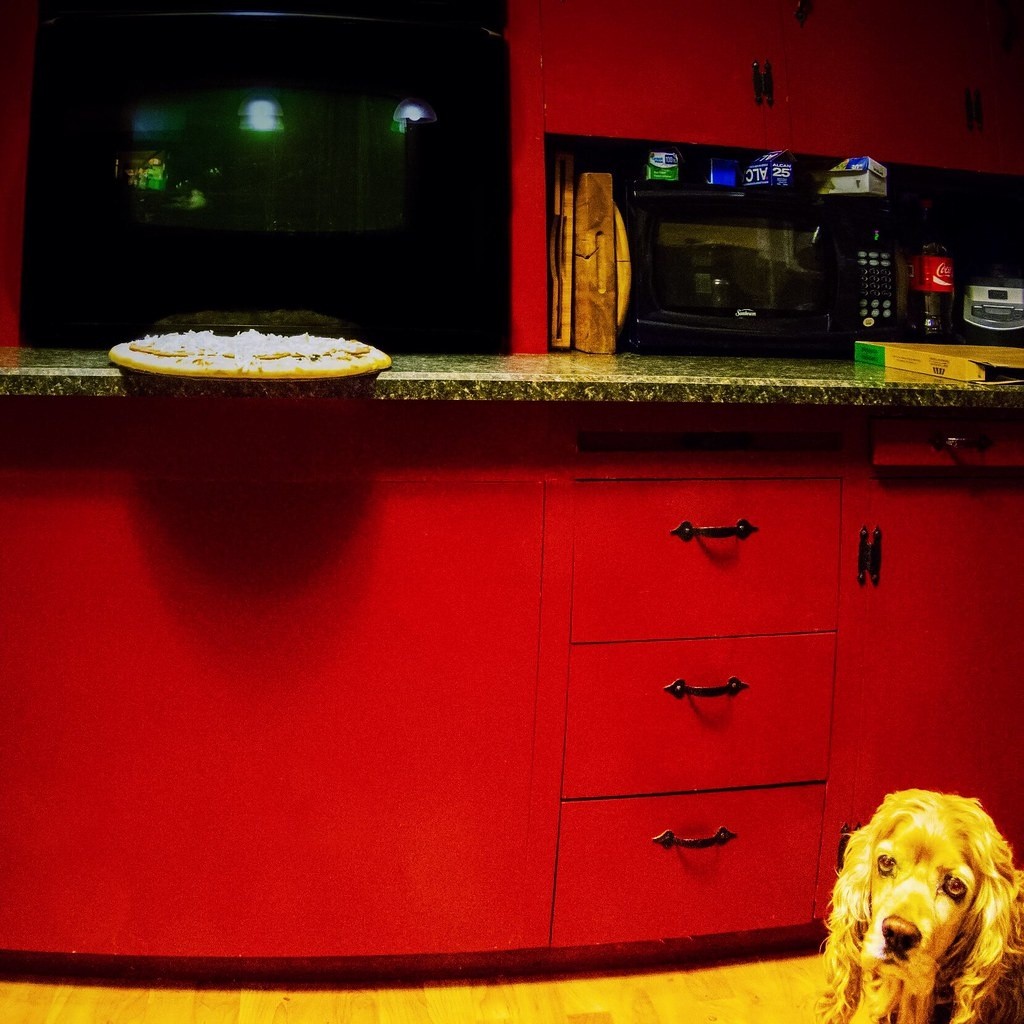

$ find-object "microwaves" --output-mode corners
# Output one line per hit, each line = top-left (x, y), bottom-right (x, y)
(615, 172), (901, 359)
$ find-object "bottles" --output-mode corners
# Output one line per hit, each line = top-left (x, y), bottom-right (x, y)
(906, 195), (959, 339)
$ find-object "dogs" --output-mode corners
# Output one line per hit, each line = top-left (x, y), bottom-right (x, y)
(811, 788), (1024, 1024)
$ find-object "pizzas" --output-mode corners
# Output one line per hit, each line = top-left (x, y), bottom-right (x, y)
(107, 328), (392, 383)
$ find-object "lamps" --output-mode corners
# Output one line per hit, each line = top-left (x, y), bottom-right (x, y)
(391, 97), (437, 132)
(237, 89), (285, 131)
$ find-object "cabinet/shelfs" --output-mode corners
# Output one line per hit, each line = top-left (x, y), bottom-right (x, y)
(540, 0), (1023, 175)
(0, 397), (1023, 975)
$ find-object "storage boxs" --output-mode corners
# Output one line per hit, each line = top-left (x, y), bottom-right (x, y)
(800, 156), (886, 198)
(643, 151), (678, 181)
(703, 158), (738, 185)
(742, 149), (799, 188)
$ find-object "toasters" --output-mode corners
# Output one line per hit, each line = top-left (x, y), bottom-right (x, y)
(959, 275), (1024, 336)
